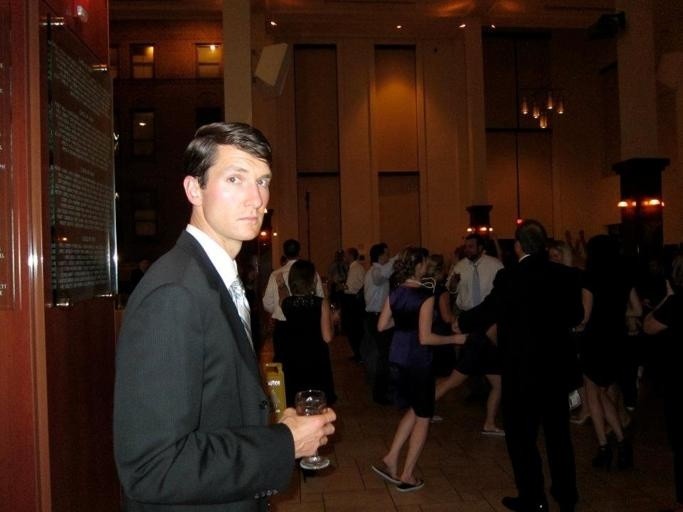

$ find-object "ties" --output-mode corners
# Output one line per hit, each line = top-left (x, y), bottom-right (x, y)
(472, 264), (481, 307)
(230, 276), (254, 348)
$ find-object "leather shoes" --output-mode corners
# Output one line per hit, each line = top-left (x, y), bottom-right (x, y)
(502, 496), (548, 512)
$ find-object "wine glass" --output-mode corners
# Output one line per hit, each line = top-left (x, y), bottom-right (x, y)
(294, 389), (333, 470)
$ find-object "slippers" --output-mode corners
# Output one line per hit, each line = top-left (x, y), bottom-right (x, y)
(396, 479), (424, 492)
(371, 464), (400, 484)
(482, 428), (506, 436)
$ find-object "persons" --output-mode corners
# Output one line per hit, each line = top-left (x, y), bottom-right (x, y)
(126, 259), (148, 290)
(114, 123), (337, 512)
